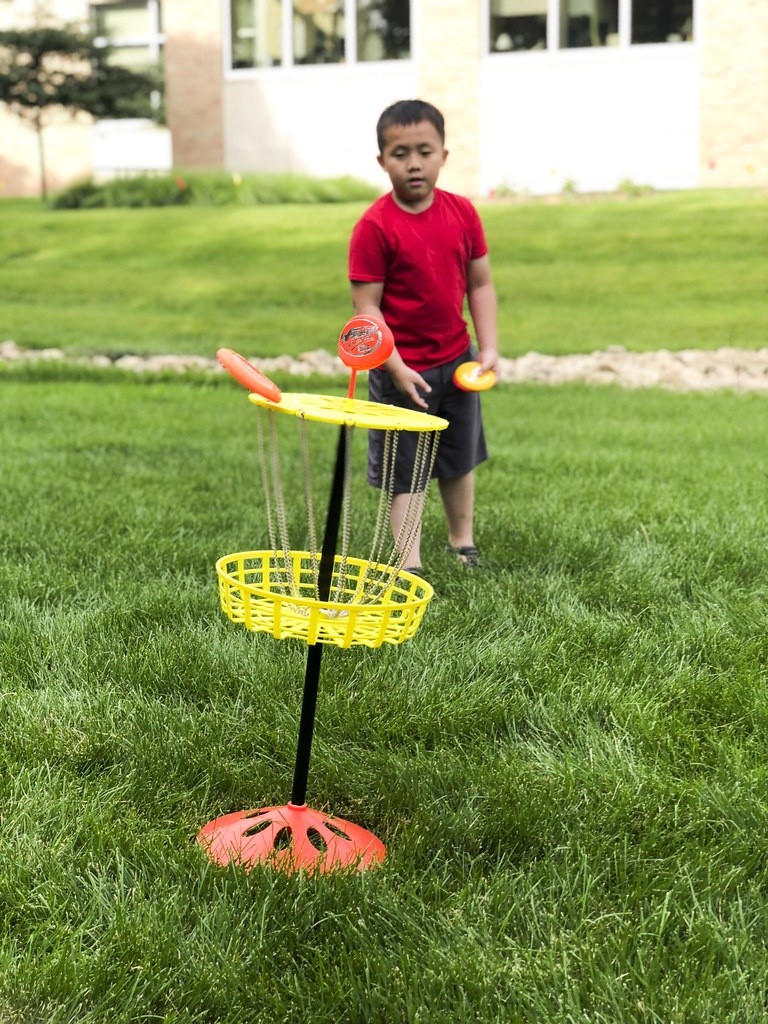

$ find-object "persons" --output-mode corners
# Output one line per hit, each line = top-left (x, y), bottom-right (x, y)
(348, 100), (501, 578)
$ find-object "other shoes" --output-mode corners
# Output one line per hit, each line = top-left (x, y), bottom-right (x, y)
(455, 547), (480, 564)
